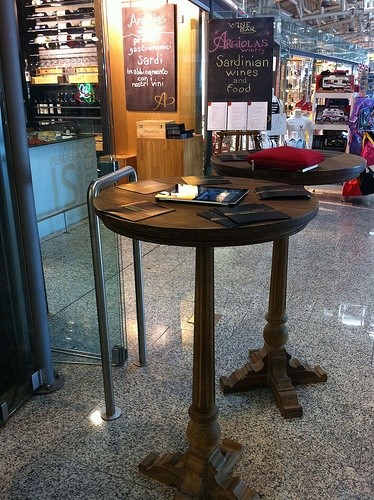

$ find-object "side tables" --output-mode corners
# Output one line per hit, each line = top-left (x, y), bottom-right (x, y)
(99, 155), (137, 186)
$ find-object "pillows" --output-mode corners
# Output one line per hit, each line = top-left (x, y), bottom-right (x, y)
(245, 146), (325, 170)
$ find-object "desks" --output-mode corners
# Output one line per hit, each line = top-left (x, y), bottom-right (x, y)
(216, 132), (250, 154)
(94, 174), (319, 500)
(135, 134), (203, 180)
(210, 150), (367, 419)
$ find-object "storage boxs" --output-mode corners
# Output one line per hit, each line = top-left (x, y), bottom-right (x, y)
(137, 120), (175, 140)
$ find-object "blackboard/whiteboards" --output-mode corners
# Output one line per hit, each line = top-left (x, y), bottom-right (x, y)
(208, 17), (274, 102)
(121, 3), (176, 111)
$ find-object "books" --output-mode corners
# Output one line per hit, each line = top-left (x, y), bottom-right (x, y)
(254, 185), (311, 201)
(196, 202), (291, 229)
(101, 199), (176, 223)
(182, 176), (231, 185)
(117, 180), (173, 194)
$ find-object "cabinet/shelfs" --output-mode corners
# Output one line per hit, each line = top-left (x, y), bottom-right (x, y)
(311, 93), (361, 153)
(17, 0), (110, 157)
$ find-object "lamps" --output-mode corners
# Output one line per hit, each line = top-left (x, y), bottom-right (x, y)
(349, 23), (354, 31)
(320, 0), (332, 7)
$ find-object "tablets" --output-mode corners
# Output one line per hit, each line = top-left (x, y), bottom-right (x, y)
(154, 183), (250, 207)
